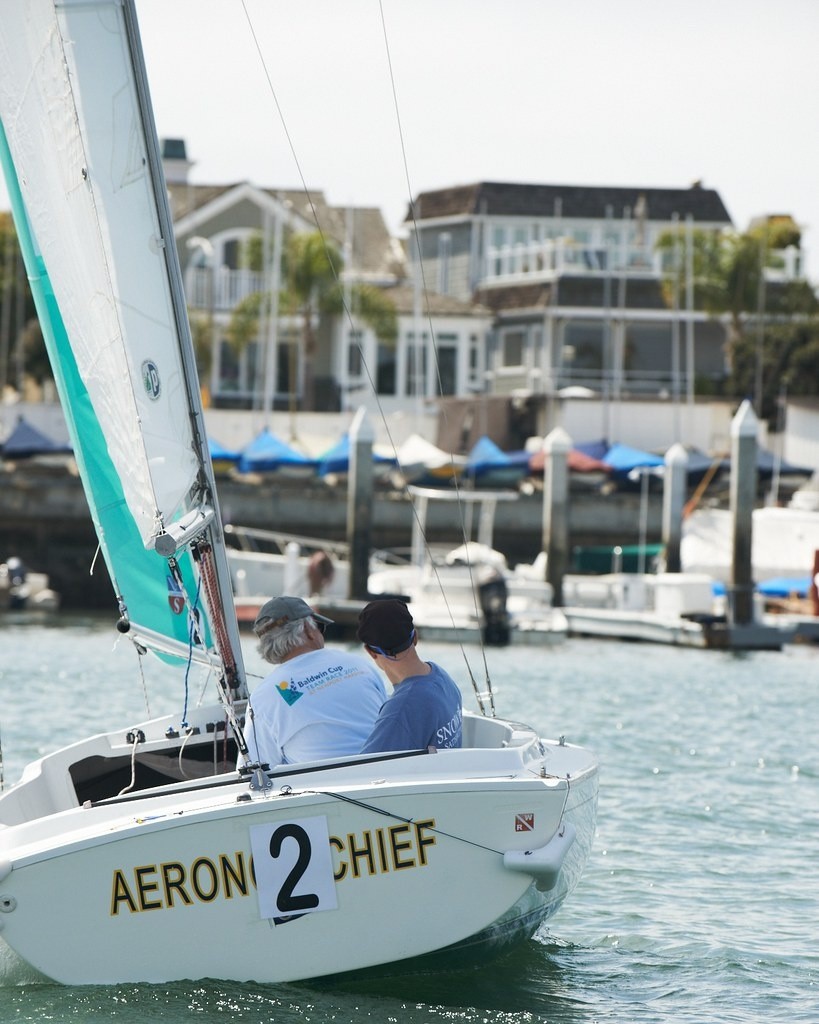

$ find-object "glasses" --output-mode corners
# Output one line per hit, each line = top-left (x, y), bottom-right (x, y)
(308, 617), (327, 634)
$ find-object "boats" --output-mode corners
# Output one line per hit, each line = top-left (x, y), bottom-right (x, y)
(223, 523), (567, 646)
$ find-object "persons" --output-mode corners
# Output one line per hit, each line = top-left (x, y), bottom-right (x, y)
(358, 599), (463, 755)
(236, 595), (388, 773)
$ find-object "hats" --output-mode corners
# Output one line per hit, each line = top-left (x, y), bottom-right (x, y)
(358, 599), (414, 656)
(254, 595), (334, 634)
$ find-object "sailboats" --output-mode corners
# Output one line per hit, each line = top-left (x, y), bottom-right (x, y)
(0, 0), (601, 987)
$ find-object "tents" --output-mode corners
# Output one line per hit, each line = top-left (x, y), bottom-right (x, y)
(209, 427), (464, 478)
(467, 436), (814, 478)
(0, 417), (71, 456)
